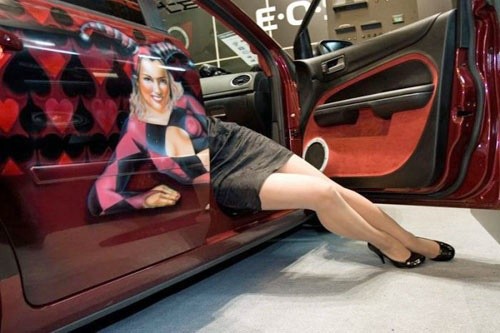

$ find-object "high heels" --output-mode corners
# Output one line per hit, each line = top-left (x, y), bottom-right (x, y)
(367, 236), (456, 268)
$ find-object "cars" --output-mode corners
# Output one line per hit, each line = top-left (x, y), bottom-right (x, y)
(0, 1), (498, 333)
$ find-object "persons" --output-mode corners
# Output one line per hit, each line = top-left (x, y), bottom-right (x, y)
(207, 116), (454, 269)
(88, 53), (210, 218)
